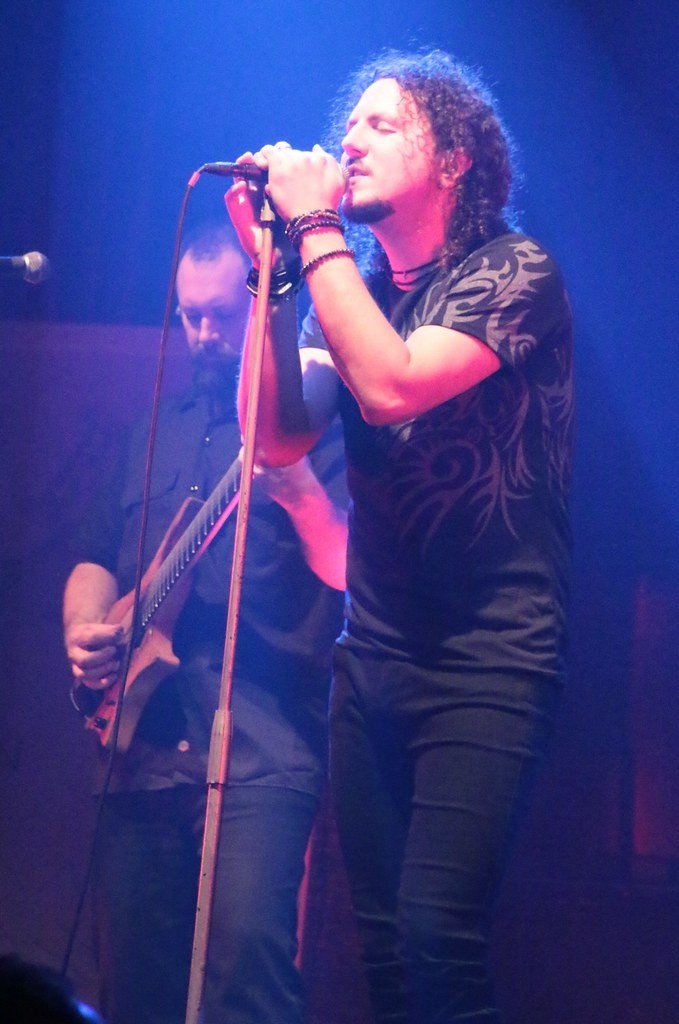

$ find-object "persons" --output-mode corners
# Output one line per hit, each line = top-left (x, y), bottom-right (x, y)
(59, 220), (367, 1024)
(225, 46), (569, 1023)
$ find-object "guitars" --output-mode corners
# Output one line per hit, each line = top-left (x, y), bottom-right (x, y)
(66, 445), (258, 762)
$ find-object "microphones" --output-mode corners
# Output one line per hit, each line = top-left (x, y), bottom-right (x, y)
(205, 161), (350, 195)
(0, 251), (52, 285)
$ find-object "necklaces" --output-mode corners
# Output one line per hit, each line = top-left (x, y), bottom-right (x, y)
(391, 257), (442, 285)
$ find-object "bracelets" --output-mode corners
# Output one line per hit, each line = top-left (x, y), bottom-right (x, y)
(246, 209), (355, 305)
